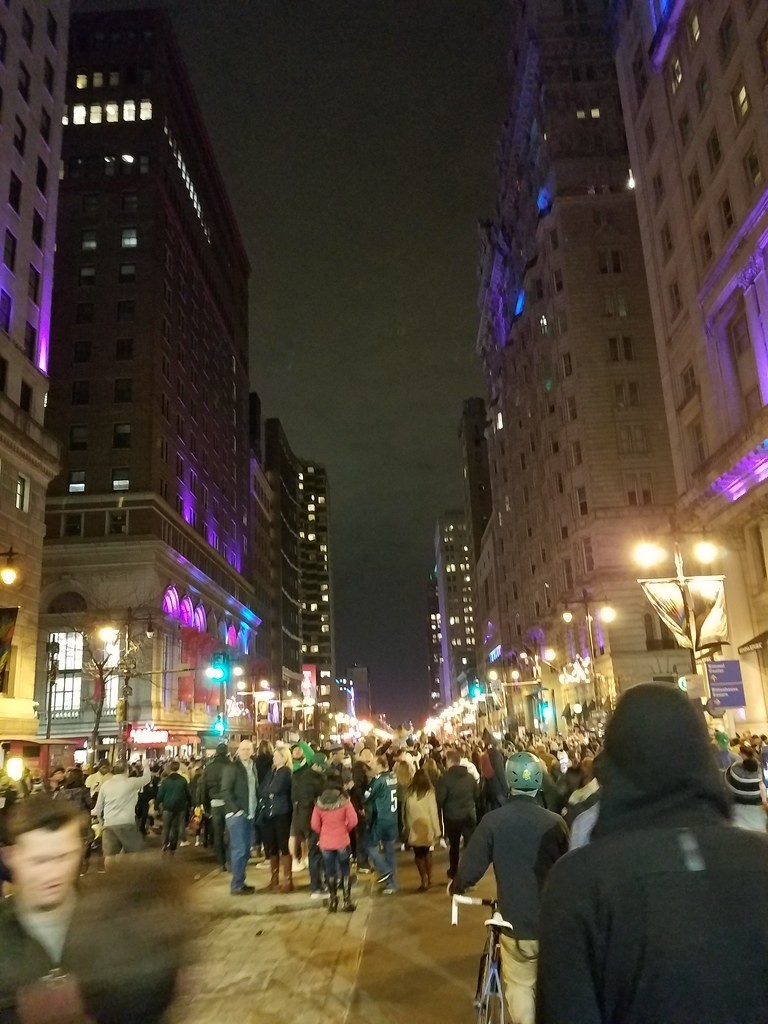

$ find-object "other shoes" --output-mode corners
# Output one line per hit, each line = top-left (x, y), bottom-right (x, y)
(429, 846), (435, 851)
(379, 888), (397, 895)
(230, 887), (256, 895)
(439, 838), (448, 849)
(377, 871), (393, 884)
(219, 865), (226, 874)
(79, 823), (213, 877)
(469, 884), (478, 891)
(447, 868), (456, 882)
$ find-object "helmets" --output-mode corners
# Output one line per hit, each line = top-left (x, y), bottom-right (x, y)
(504, 752), (542, 791)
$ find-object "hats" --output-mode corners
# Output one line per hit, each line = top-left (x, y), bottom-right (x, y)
(725, 758), (764, 802)
(330, 743), (345, 755)
(311, 753), (331, 770)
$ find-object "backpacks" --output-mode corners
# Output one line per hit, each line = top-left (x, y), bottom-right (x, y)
(479, 752), (495, 780)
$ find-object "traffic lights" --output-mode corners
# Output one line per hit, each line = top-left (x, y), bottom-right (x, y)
(210, 654), (229, 681)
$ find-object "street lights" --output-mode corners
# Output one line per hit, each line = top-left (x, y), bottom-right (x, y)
(98, 607), (157, 768)
(634, 505), (731, 703)
(565, 591), (615, 711)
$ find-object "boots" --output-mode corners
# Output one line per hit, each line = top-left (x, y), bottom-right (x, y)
(414, 856), (429, 892)
(425, 852), (433, 888)
(341, 875), (354, 912)
(280, 855), (295, 893)
(325, 876), (339, 912)
(258, 855), (281, 894)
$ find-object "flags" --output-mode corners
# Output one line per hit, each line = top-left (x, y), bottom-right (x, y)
(687, 580), (730, 651)
(642, 582), (692, 651)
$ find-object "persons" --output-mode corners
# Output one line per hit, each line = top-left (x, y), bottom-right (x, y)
(310, 774), (357, 913)
(3, 714), (767, 880)
(254, 746), (295, 893)
(401, 768), (441, 894)
(724, 758), (767, 833)
(448, 752), (569, 1024)
(298, 752), (331, 900)
(361, 756), (399, 894)
(534, 682), (767, 1024)
(1, 793), (191, 1023)
(220, 739), (260, 896)
